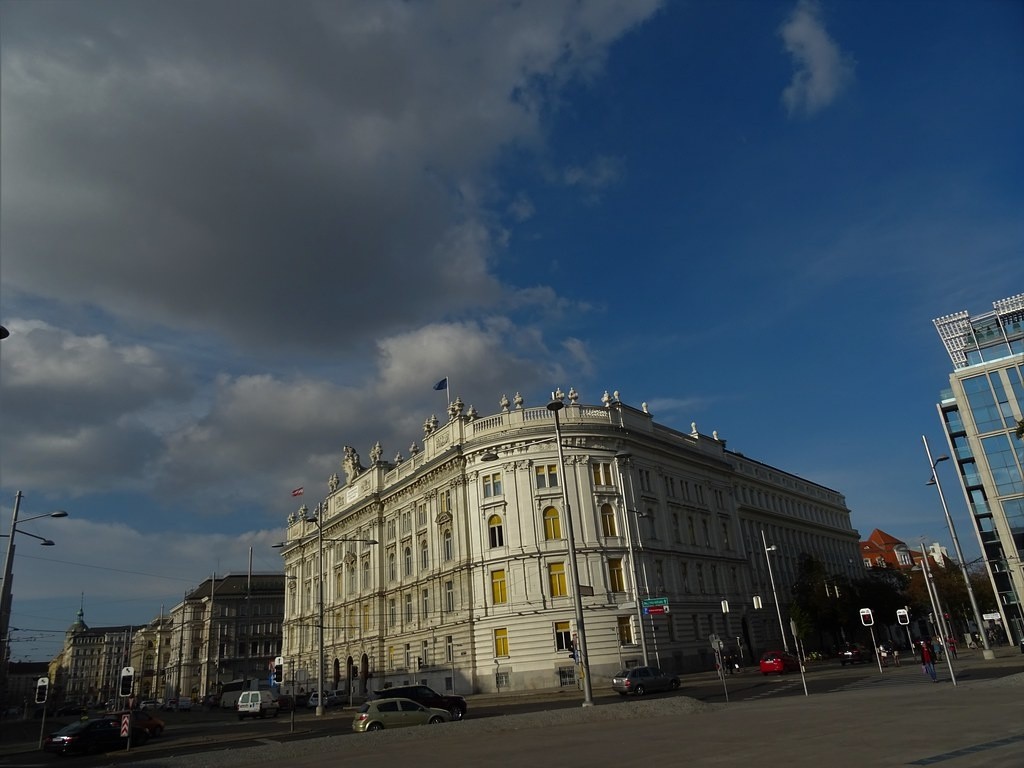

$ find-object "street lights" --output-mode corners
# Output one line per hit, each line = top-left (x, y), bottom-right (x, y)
(0, 509), (69, 663)
(625, 508), (649, 665)
(926, 455), (996, 660)
(764, 544), (789, 652)
(271, 515), (378, 716)
(546, 400), (596, 707)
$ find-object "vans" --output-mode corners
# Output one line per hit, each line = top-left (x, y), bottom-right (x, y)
(236, 691), (280, 720)
(306, 690), (349, 707)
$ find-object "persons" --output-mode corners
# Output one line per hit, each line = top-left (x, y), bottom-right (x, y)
(715, 654), (741, 680)
(193, 695), (219, 711)
(811, 650), (826, 665)
(877, 624), (1003, 683)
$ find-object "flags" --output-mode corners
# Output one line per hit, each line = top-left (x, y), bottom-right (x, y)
(433, 378), (448, 390)
(291, 487), (304, 497)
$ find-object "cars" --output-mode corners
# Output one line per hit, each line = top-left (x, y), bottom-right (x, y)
(35, 697), (191, 754)
(351, 698), (452, 732)
(760, 652), (800, 675)
(839, 645), (872, 665)
(612, 665), (681, 696)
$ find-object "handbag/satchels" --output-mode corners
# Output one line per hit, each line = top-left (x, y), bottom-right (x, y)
(734, 663), (740, 669)
(921, 663), (928, 677)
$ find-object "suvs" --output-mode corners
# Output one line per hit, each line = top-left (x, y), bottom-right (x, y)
(373, 685), (467, 722)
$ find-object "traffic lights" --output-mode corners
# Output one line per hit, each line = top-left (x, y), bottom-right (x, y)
(860, 608), (874, 626)
(35, 677), (49, 703)
(568, 640), (575, 659)
(119, 667), (134, 697)
(896, 609), (909, 625)
(418, 657), (422, 670)
(275, 657), (283, 682)
